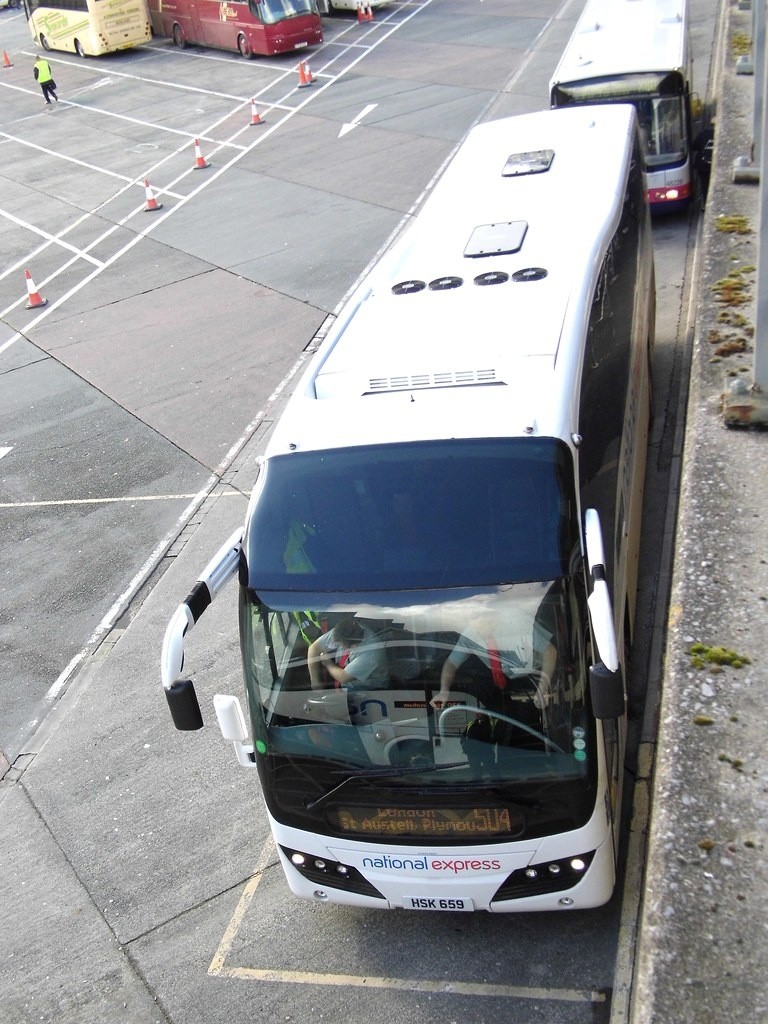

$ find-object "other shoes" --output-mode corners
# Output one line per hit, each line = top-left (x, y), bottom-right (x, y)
(55, 97), (58, 101)
(44, 101), (50, 104)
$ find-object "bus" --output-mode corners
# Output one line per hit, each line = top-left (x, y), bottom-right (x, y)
(548, 0), (704, 212)
(158, 102), (652, 919)
(20, 0), (152, 59)
(323, 0), (396, 17)
(150, 0), (325, 59)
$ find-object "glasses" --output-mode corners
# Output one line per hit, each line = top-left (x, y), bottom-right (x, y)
(468, 610), (492, 620)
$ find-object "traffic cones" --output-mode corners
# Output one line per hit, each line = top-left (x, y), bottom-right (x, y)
(297, 62), (312, 88)
(367, 2), (375, 21)
(249, 99), (265, 125)
(192, 138), (211, 170)
(360, 1), (367, 20)
(23, 268), (48, 310)
(143, 179), (164, 212)
(356, 6), (366, 23)
(303, 60), (318, 83)
(3, 50), (14, 68)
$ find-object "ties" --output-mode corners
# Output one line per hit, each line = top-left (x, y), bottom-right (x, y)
(487, 638), (507, 690)
(335, 650), (350, 688)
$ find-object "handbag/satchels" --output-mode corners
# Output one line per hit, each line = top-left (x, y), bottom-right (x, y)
(48, 79), (56, 90)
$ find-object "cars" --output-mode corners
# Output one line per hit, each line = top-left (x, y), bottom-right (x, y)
(0, 0), (21, 8)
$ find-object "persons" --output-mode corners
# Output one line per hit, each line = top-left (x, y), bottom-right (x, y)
(307, 617), (390, 690)
(428, 602), (558, 752)
(34, 55), (58, 104)
